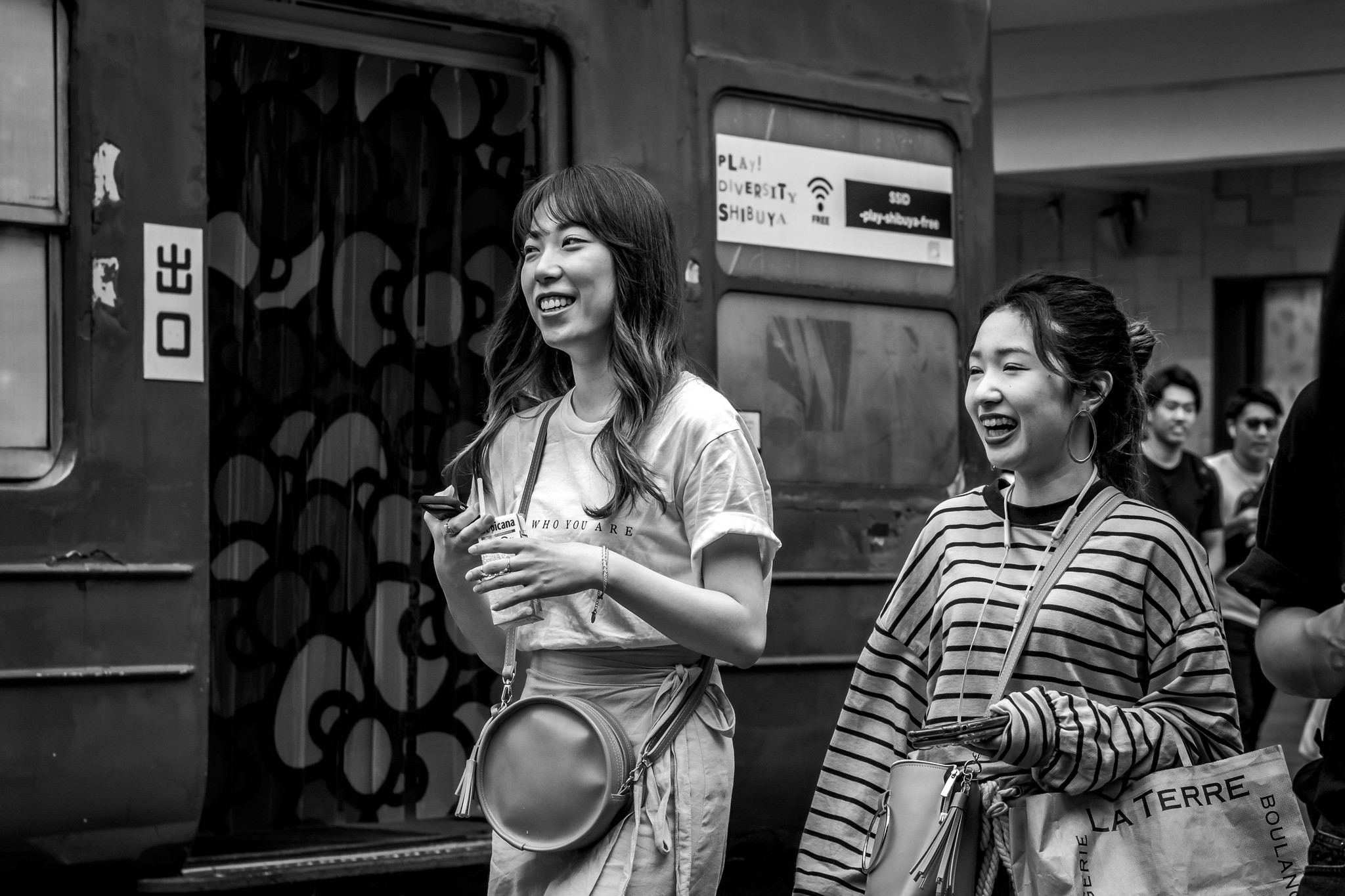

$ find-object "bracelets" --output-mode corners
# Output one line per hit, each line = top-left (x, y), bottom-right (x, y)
(591, 545), (609, 623)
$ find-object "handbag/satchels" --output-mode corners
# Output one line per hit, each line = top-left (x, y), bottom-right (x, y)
(454, 691), (636, 858)
(1006, 712), (1311, 896)
(860, 760), (1030, 895)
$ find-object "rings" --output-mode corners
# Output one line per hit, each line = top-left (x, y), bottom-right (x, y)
(479, 554), (511, 578)
(443, 517), (457, 536)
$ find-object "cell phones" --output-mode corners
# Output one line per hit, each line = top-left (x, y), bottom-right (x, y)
(909, 715), (1011, 749)
(418, 495), (481, 524)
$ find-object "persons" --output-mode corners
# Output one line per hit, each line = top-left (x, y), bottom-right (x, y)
(1102, 364), (1226, 618)
(1201, 381), (1285, 751)
(1226, 204), (1345, 896)
(420, 163), (783, 896)
(789, 272), (1245, 896)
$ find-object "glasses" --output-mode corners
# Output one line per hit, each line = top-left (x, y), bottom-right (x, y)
(1240, 417), (1277, 432)
